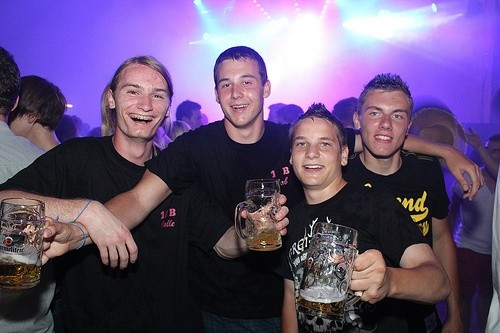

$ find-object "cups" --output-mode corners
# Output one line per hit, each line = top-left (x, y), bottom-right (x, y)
(235, 179), (282, 252)
(297, 222), (363, 320)
(0, 198), (45, 289)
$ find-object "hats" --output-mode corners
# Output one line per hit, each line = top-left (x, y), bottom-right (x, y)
(408, 108), (467, 166)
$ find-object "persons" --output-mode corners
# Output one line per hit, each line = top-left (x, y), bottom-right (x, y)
(23, 46), (485, 333)
(7, 75), (68, 152)
(164, 100), (208, 142)
(0, 46), (57, 333)
(457, 127), (500, 333)
(341, 72), (466, 333)
(268, 97), (359, 129)
(270, 102), (452, 333)
(0, 55), (290, 333)
(55, 114), (102, 144)
(447, 135), (500, 333)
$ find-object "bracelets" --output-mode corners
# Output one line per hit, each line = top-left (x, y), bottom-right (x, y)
(66, 223), (86, 249)
(73, 200), (93, 223)
